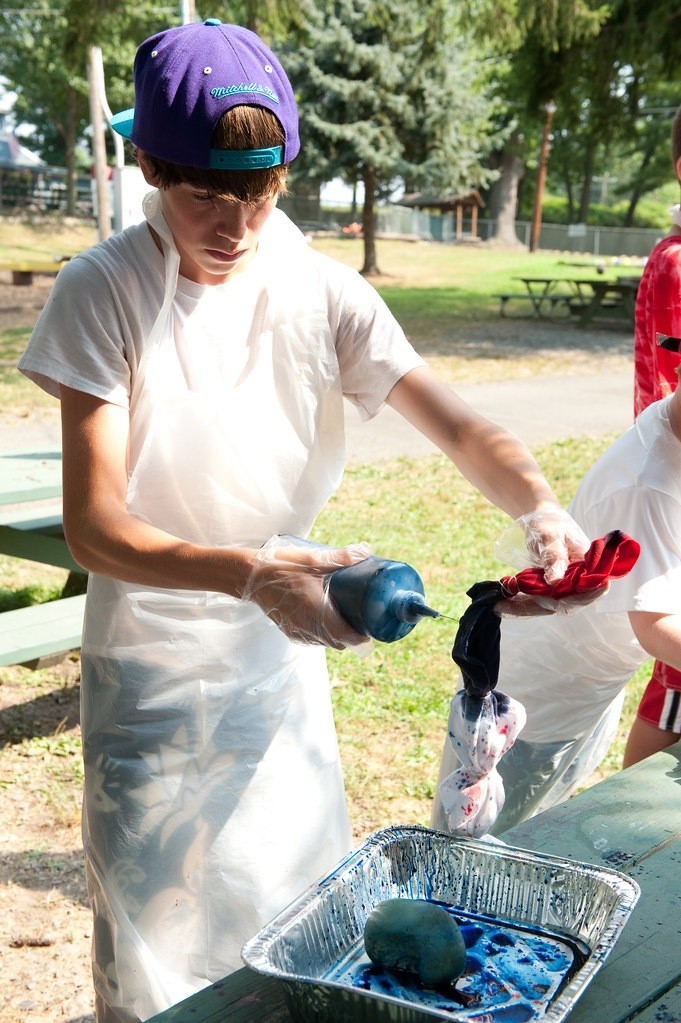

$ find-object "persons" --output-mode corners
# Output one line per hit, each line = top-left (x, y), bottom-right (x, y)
(19, 23), (607, 1023)
(439, 366), (681, 841)
(624, 102), (681, 766)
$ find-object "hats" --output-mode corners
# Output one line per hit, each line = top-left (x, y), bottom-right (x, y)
(109, 18), (302, 170)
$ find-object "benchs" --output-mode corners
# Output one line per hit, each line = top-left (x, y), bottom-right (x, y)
(0, 261), (66, 287)
(1, 593), (89, 666)
(493, 293), (575, 318)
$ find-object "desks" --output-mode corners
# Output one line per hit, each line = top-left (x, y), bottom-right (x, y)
(0, 427), (110, 595)
(151, 732), (681, 1021)
(514, 278), (639, 327)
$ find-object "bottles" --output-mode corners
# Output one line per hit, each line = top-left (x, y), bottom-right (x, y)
(263, 534), (441, 644)
